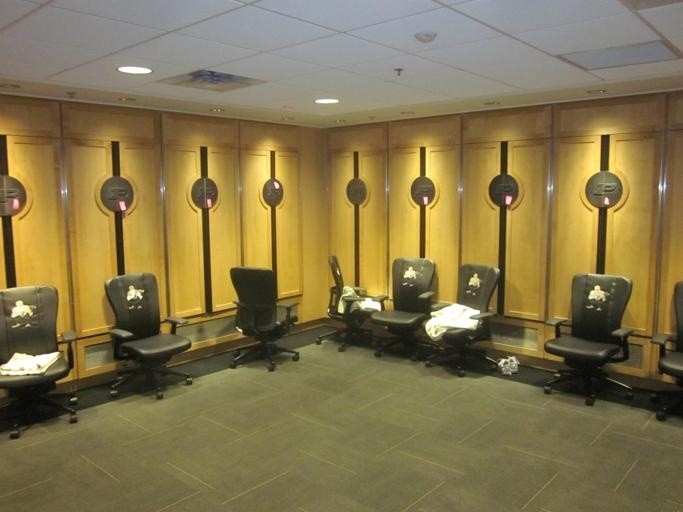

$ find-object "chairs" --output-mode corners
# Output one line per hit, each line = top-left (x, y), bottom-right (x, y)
(316, 256), (390, 353)
(103, 271), (193, 400)
(421, 262), (501, 377)
(228, 269), (301, 373)
(1, 284), (76, 438)
(543, 272), (635, 406)
(649, 281), (683, 424)
(369, 258), (436, 361)
